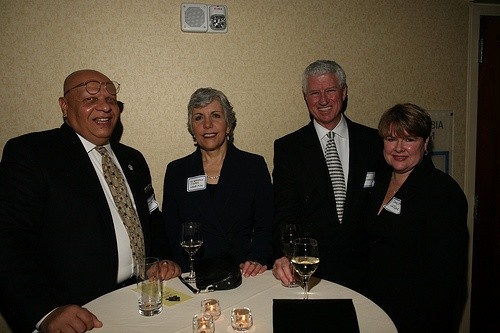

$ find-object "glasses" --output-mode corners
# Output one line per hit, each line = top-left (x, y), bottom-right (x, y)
(64, 81), (120, 94)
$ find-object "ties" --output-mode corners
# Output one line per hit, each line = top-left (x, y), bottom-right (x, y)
(96, 146), (145, 280)
(325, 131), (347, 223)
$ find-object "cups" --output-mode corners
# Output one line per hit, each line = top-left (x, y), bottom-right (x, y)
(201, 298), (221, 321)
(192, 312), (215, 333)
(136, 256), (163, 317)
(230, 307), (254, 333)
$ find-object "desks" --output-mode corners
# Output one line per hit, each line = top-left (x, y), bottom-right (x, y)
(81, 271), (399, 333)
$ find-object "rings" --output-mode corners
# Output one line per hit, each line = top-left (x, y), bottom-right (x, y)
(251, 262), (256, 265)
(161, 261), (168, 265)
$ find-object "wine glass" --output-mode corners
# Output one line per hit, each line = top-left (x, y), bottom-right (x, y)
(281, 224), (301, 288)
(290, 237), (320, 299)
(180, 222), (204, 283)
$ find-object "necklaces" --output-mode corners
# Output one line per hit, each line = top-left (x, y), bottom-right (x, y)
(205, 172), (220, 180)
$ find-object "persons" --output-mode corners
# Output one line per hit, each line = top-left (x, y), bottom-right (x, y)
(0, 69), (162, 333)
(269, 60), (393, 289)
(158, 88), (274, 279)
(342, 104), (471, 333)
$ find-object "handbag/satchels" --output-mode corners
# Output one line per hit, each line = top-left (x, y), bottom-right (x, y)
(195, 254), (242, 292)
